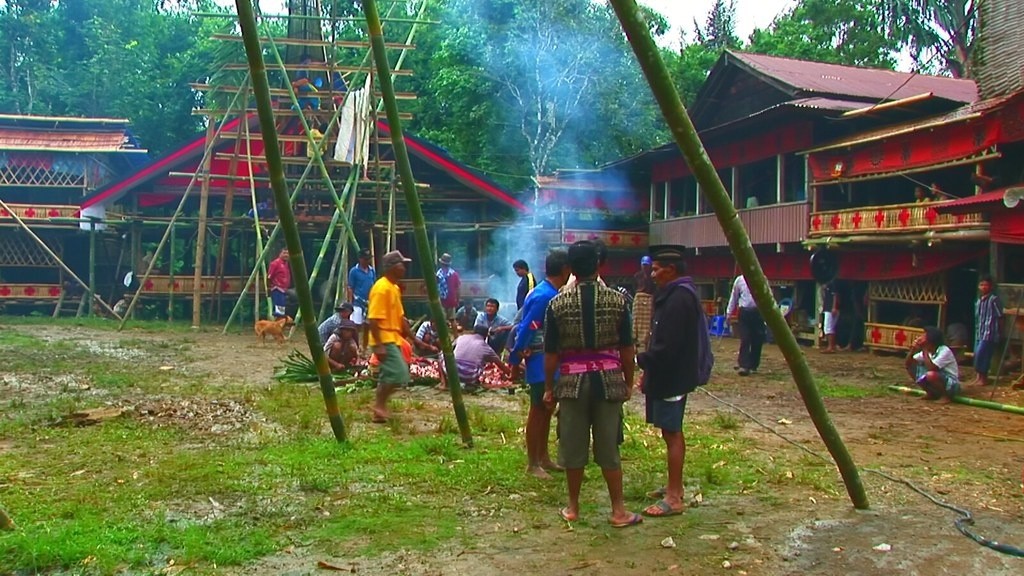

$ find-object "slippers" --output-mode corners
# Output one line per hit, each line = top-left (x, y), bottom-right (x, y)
(641, 501), (684, 516)
(646, 489), (683, 499)
(558, 507), (575, 521)
(611, 514), (643, 527)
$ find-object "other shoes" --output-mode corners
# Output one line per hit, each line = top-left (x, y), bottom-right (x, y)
(738, 367), (749, 374)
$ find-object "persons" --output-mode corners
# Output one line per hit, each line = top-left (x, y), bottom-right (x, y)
(266, 241), (766, 526)
(914, 182), (948, 204)
(824, 283), (841, 353)
(966, 277), (1004, 386)
(905, 326), (960, 403)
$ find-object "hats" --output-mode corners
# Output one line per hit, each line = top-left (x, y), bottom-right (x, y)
(641, 255), (651, 265)
(335, 302), (354, 312)
(357, 248), (373, 259)
(439, 253), (452, 265)
(339, 321), (356, 329)
(382, 250), (412, 267)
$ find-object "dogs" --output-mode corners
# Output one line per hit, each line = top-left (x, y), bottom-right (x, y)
(254, 314), (296, 348)
